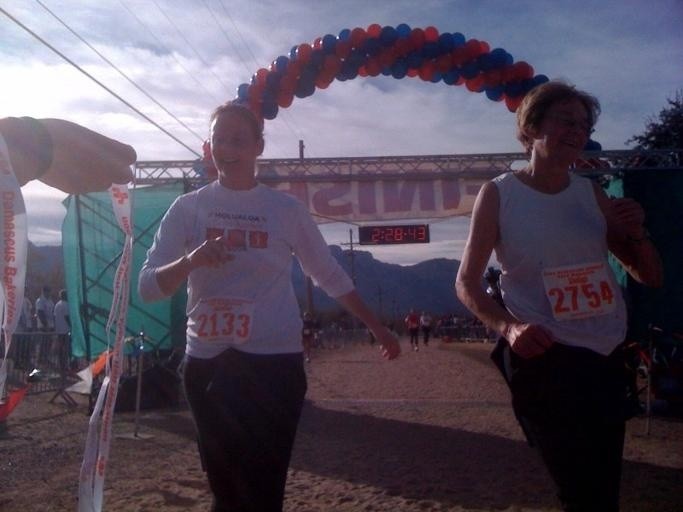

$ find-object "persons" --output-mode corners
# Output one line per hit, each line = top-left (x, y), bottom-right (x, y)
(404, 308), (420, 352)
(15, 284), (71, 369)
(136, 102), (401, 511)
(454, 81), (660, 512)
(302, 312), (315, 363)
(0, 115), (136, 195)
(420, 312), (432, 346)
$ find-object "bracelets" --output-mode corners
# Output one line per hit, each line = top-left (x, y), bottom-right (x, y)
(21, 115), (56, 180)
(624, 228), (652, 246)
